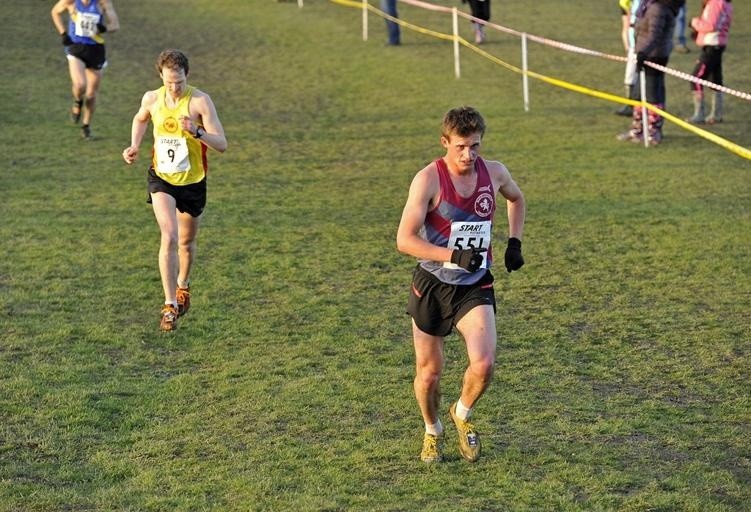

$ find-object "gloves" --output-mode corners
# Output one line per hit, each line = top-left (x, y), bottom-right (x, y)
(634, 52), (646, 72)
(504, 237), (524, 272)
(96, 22), (106, 32)
(450, 247), (486, 272)
(61, 31), (73, 45)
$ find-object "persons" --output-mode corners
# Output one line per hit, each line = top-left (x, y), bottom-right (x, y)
(614, 0), (642, 118)
(462, 1), (490, 46)
(684, 0), (733, 124)
(616, 0), (685, 146)
(673, 5), (690, 54)
(122, 49), (228, 331)
(395, 107), (525, 463)
(381, 0), (404, 49)
(50, 0), (120, 139)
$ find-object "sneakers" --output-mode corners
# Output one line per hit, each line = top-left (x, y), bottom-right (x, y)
(176, 283), (191, 315)
(160, 304), (178, 332)
(72, 100), (81, 124)
(450, 402), (482, 462)
(80, 125), (91, 137)
(420, 431), (445, 463)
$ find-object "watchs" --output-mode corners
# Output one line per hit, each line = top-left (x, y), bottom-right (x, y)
(192, 123), (205, 139)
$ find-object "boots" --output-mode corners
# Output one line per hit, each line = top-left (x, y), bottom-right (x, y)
(647, 104), (665, 145)
(704, 91), (722, 124)
(615, 85), (640, 116)
(617, 106), (642, 143)
(686, 90), (704, 123)
(473, 23), (483, 42)
